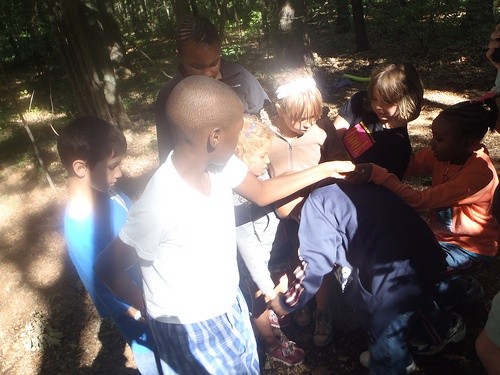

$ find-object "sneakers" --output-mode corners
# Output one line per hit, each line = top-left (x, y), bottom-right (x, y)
(266, 339), (306, 366)
(312, 307), (334, 348)
(269, 309), (291, 328)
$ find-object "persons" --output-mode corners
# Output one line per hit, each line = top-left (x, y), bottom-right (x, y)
(153, 13), (500, 375)
(56, 115), (160, 375)
(92, 75), (364, 375)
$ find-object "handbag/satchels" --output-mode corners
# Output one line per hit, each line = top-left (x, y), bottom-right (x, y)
(404, 312), (487, 375)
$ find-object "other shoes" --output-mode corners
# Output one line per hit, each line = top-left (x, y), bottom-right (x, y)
(359, 350), (417, 373)
(294, 303), (312, 327)
